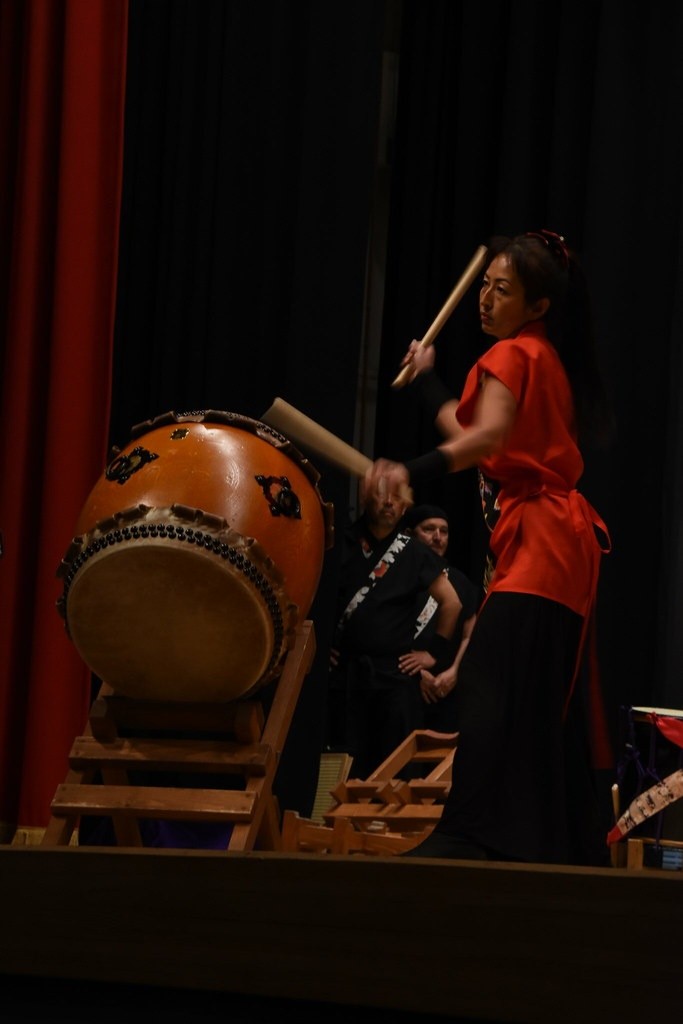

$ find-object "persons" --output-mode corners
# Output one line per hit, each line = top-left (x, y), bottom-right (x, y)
(399, 502), (478, 731)
(317, 458), (464, 778)
(353, 230), (603, 867)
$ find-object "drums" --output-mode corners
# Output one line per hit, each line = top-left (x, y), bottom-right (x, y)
(52, 405), (342, 717)
